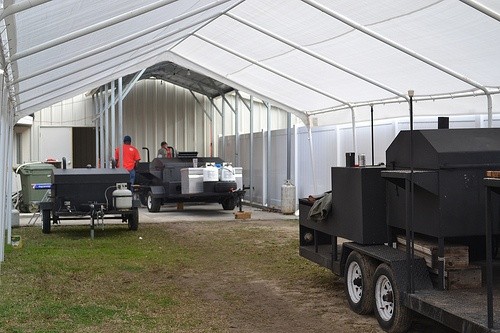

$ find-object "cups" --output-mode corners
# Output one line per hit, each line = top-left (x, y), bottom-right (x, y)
(193, 158), (198, 168)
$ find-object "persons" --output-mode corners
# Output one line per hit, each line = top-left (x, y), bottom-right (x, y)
(158, 141), (167, 157)
(114, 136), (141, 194)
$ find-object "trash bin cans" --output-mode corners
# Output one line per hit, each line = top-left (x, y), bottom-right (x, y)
(12, 162), (56, 214)
